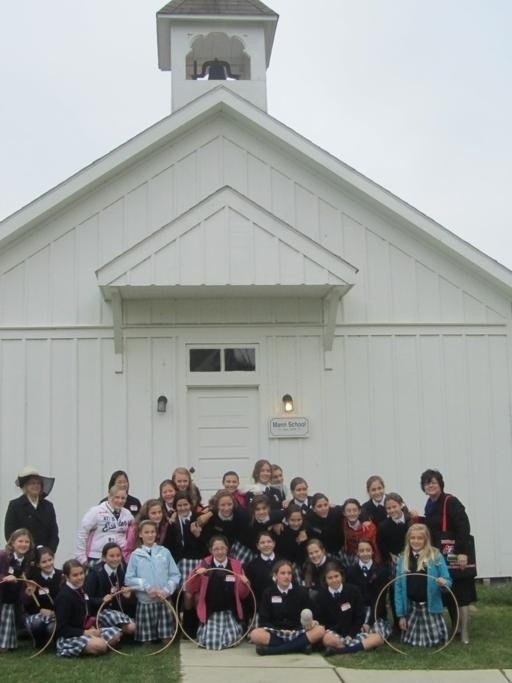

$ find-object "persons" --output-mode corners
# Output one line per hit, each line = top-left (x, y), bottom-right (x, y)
(393, 524), (452, 647)
(5, 465), (59, 553)
(1, 528), (63, 651)
(56, 558), (121, 656)
(76, 460), (415, 658)
(408, 470), (476, 644)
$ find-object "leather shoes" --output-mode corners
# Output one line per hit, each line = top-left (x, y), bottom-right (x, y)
(325, 646), (336, 657)
(255, 644), (267, 655)
(461, 639), (473, 645)
(304, 643), (314, 655)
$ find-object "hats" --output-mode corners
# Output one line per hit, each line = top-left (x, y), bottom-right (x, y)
(14, 466), (55, 499)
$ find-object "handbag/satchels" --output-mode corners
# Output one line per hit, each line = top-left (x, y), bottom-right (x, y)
(434, 531), (477, 579)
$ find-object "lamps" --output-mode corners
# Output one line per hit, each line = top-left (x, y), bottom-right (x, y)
(282, 392), (293, 412)
(155, 395), (169, 412)
